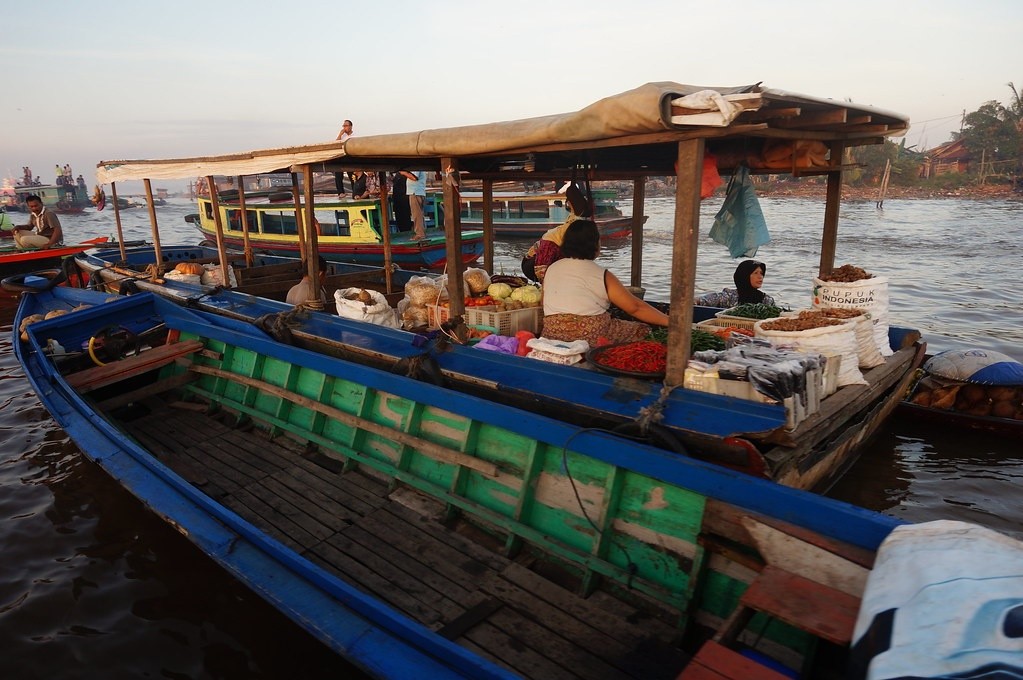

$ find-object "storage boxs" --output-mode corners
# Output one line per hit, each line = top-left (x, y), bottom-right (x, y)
(465, 302), (541, 334)
(423, 301), (449, 331)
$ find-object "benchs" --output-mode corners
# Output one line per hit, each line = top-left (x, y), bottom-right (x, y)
(64, 339), (205, 393)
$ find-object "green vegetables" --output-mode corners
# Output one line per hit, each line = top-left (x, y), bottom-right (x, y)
(722, 302), (790, 320)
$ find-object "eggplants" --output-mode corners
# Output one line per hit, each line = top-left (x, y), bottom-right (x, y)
(490, 274), (529, 288)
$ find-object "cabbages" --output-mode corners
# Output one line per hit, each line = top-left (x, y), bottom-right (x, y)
(488, 282), (542, 303)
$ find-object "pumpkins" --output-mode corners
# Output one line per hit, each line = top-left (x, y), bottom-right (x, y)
(175, 262), (205, 276)
(20, 296), (120, 341)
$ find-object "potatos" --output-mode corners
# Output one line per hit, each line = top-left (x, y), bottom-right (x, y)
(485, 301), (542, 313)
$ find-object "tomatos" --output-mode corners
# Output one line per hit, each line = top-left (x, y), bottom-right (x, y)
(431, 295), (501, 325)
(714, 325), (754, 339)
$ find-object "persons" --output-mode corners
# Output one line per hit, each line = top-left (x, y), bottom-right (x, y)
(0, 208), (14, 231)
(361, 172), (391, 199)
(11, 196), (63, 249)
(540, 220), (670, 346)
(400, 170), (427, 240)
(519, 222), (577, 280)
(285, 256), (327, 304)
(22, 166), (41, 185)
(334, 120), (355, 197)
(351, 171), (369, 198)
(390, 170), (413, 232)
(54, 164), (84, 186)
(693, 260), (776, 308)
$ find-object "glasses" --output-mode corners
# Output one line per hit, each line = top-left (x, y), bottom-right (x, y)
(342, 125), (351, 128)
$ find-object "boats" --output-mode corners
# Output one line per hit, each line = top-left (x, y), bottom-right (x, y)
(1, 82), (1023, 502)
(10, 273), (1022, 680)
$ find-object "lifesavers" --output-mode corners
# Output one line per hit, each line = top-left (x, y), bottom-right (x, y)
(268, 192), (294, 201)
(57, 202), (72, 210)
(198, 240), (218, 248)
(0, 268), (65, 291)
(219, 190), (238, 196)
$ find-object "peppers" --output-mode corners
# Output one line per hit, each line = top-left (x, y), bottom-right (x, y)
(596, 326), (727, 373)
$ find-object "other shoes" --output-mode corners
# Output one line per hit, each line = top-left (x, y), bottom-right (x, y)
(409, 235), (424, 241)
(339, 193), (346, 200)
